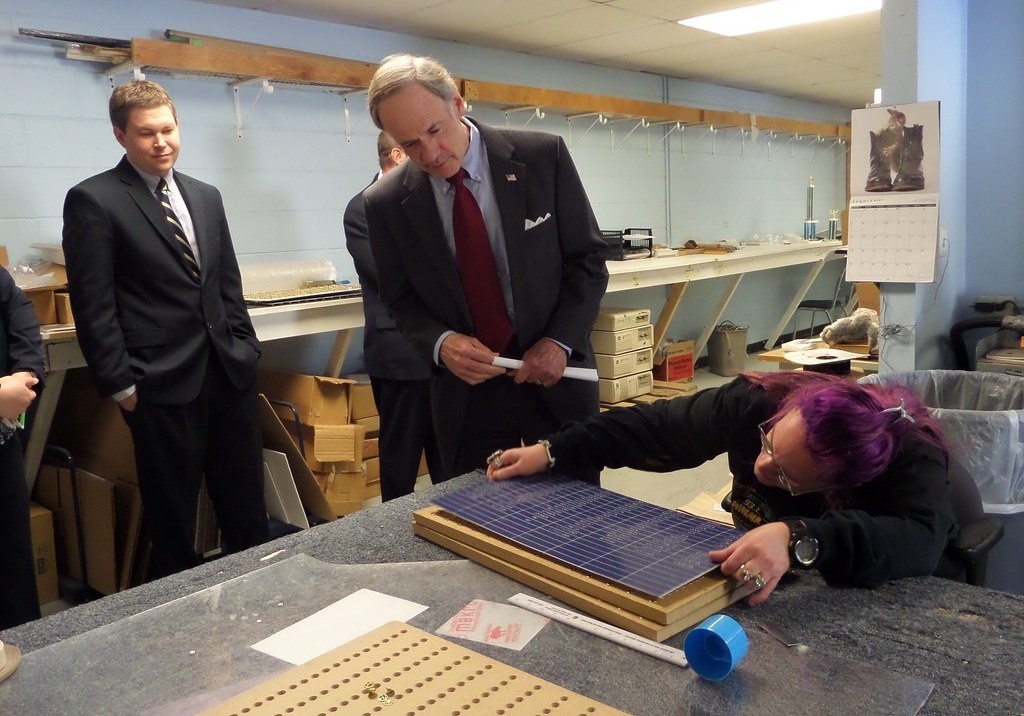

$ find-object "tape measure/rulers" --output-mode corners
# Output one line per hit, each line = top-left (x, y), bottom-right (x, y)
(507, 592), (689, 668)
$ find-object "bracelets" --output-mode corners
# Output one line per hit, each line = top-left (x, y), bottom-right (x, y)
(537, 438), (555, 475)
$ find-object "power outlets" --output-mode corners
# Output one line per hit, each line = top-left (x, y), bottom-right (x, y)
(977, 296), (1014, 318)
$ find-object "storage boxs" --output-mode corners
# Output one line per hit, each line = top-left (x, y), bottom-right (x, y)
(11, 254), (694, 603)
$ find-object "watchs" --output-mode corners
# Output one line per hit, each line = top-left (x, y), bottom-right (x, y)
(782, 519), (818, 571)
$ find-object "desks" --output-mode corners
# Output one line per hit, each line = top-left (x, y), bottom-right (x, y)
(26, 243), (846, 498)
(0, 464), (1024, 716)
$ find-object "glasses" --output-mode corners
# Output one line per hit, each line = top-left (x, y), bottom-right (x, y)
(757, 413), (838, 497)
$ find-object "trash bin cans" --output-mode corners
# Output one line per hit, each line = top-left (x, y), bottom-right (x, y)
(707, 326), (747, 376)
(853, 369), (1024, 593)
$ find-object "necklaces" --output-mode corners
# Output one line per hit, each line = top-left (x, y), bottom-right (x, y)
(25, 370), (36, 380)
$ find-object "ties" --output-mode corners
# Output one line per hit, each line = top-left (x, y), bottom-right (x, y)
(444, 168), (515, 357)
(156, 179), (202, 282)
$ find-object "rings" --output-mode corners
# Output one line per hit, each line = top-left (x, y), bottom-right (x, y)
(741, 565), (767, 588)
(487, 448), (503, 468)
(535, 379), (543, 385)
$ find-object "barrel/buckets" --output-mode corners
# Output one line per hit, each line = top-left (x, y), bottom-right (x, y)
(707, 321), (749, 377)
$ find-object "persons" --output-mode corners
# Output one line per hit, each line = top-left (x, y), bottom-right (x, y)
(363, 52), (612, 491)
(0, 262), (48, 633)
(344, 129), (469, 505)
(62, 80), (269, 584)
(488, 370), (986, 607)
